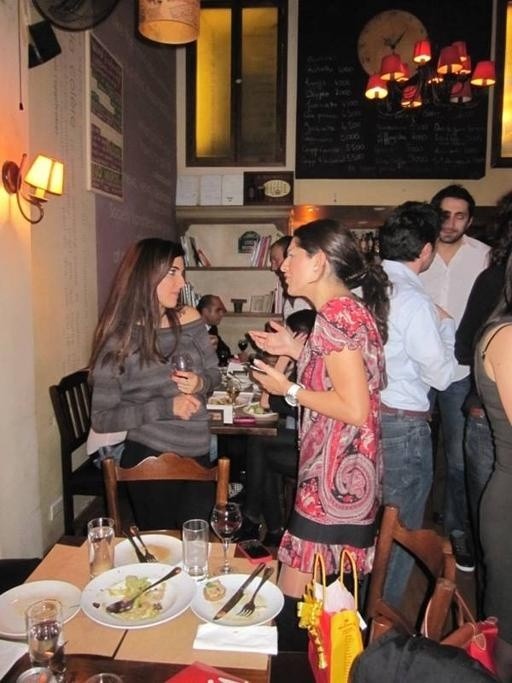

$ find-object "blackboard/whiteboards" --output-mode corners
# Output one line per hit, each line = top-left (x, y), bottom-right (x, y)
(85, 30), (124, 203)
(295, 0), (493, 180)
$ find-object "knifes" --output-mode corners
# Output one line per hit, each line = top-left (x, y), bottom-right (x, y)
(121, 525), (148, 564)
(213, 561), (266, 620)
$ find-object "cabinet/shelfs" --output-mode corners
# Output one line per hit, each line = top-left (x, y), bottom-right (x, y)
(172, 203), (293, 320)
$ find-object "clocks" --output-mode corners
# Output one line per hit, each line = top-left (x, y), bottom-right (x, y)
(352, 7), (428, 87)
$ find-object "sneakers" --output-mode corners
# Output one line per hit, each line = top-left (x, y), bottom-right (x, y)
(450, 530), (474, 573)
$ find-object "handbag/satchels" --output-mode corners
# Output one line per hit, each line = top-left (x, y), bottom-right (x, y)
(298, 549), (365, 683)
(438, 617), (499, 676)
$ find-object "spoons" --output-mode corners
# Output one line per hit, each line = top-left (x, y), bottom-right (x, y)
(106, 566), (182, 613)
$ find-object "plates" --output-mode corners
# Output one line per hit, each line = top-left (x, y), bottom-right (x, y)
(206, 393), (248, 409)
(242, 401), (280, 418)
(190, 572), (285, 629)
(1, 579), (83, 640)
(79, 562), (200, 630)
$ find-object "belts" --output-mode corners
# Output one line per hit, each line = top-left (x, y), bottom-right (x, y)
(470, 408), (485, 419)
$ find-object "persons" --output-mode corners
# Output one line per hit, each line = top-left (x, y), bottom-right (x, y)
(87, 238), (231, 531)
(247, 184), (511, 653)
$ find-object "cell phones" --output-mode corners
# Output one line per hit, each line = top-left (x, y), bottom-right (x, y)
(245, 360), (266, 377)
(233, 416), (255, 425)
(238, 539), (273, 563)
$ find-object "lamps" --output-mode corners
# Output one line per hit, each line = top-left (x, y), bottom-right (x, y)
(4, 155), (66, 225)
(364, 35), (499, 124)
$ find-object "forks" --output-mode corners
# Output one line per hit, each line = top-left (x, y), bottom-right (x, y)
(130, 524), (157, 562)
(235, 567), (275, 618)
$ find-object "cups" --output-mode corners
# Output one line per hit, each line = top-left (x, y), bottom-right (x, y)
(183, 518), (209, 581)
(87, 516), (114, 580)
(173, 353), (195, 374)
(86, 673), (122, 683)
(16, 599), (66, 683)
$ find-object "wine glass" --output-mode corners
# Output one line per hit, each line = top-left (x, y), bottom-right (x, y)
(211, 501), (242, 575)
(237, 332), (250, 360)
(226, 378), (240, 410)
